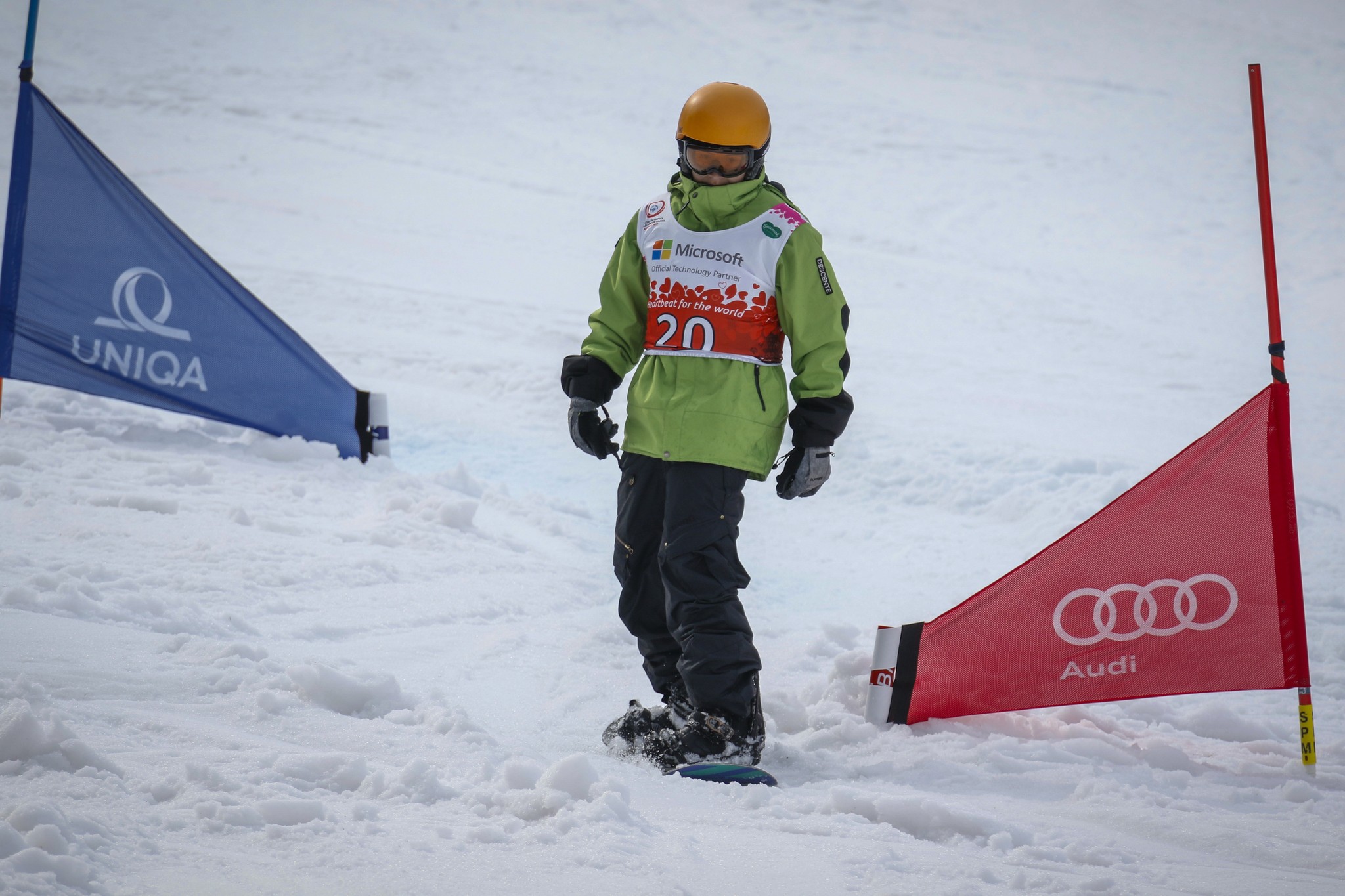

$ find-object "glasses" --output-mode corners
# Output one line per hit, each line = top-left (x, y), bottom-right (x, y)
(676, 122), (771, 177)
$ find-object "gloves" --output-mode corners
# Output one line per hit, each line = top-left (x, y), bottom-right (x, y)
(775, 444), (835, 500)
(560, 397), (622, 462)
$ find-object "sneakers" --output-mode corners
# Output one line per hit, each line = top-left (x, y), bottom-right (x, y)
(644, 711), (761, 772)
(617, 696), (694, 742)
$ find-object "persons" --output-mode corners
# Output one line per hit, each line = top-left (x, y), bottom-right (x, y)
(561, 83), (854, 776)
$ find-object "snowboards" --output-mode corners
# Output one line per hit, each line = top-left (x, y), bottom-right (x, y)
(602, 715), (778, 787)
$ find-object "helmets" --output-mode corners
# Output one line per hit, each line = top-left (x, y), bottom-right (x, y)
(675, 81), (772, 150)
